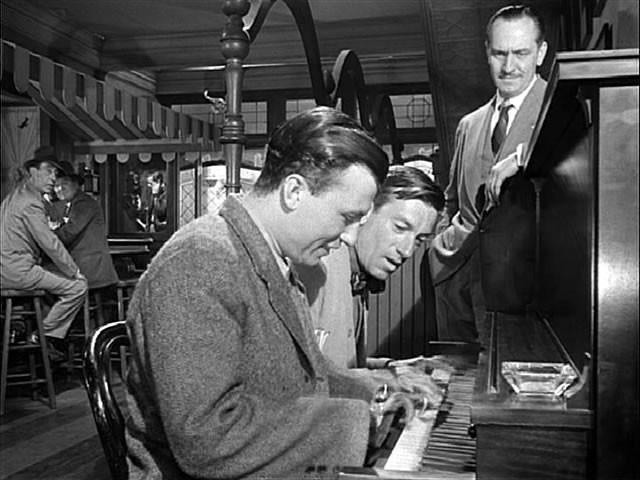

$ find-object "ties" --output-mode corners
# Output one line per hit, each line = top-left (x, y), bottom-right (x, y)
(489, 104), (515, 159)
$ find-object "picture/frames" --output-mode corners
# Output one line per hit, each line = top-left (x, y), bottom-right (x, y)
(109, 148), (176, 240)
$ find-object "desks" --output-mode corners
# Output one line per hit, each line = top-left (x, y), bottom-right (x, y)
(109, 244), (152, 259)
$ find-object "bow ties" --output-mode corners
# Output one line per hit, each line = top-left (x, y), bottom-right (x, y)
(348, 270), (372, 312)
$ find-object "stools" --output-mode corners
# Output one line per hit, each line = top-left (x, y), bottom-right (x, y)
(0, 284), (58, 414)
(61, 280), (140, 396)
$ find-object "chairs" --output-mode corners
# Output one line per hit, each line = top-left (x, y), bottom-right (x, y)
(81, 318), (147, 480)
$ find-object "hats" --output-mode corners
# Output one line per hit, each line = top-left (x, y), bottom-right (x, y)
(24, 145), (64, 176)
(55, 160), (86, 186)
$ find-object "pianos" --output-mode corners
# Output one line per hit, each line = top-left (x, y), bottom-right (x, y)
(337, 49), (639, 480)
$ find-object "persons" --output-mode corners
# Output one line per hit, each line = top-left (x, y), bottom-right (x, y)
(31, 160), (122, 344)
(289, 163), (464, 413)
(423, 4), (551, 355)
(0, 145), (88, 362)
(120, 104), (447, 480)
